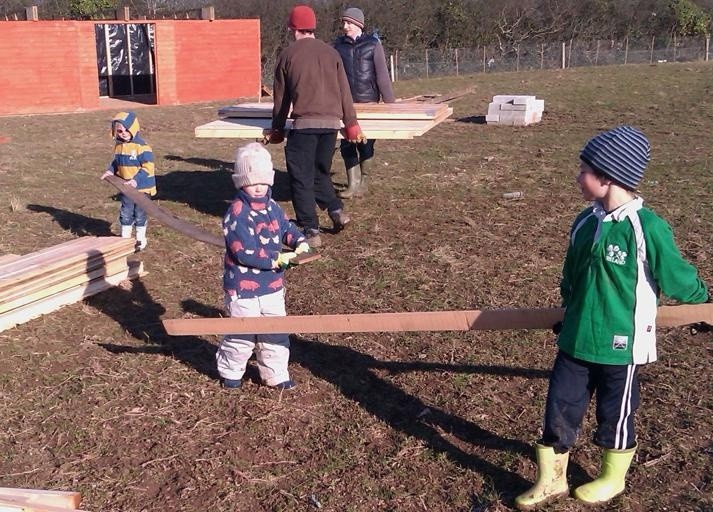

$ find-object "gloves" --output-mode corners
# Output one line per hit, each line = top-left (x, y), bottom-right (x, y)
(294, 242), (309, 254)
(278, 251), (297, 269)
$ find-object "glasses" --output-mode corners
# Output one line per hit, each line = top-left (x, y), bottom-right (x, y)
(117, 129), (128, 133)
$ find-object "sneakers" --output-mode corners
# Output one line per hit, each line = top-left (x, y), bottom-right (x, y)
(224, 379), (241, 389)
(329, 208), (351, 231)
(277, 381), (297, 390)
(304, 226), (322, 248)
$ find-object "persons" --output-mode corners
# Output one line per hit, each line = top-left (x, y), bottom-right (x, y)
(326, 8), (397, 202)
(97, 109), (158, 252)
(256, 7), (366, 242)
(215, 143), (312, 389)
(513, 128), (712, 512)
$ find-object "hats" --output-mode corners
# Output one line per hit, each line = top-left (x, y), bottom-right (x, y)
(580, 126), (650, 191)
(232, 143), (276, 189)
(288, 6), (316, 29)
(342, 8), (364, 29)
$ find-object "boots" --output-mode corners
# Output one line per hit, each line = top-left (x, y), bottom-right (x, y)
(121, 225), (132, 238)
(135, 226), (147, 250)
(515, 440), (570, 511)
(337, 164), (361, 198)
(574, 435), (639, 503)
(354, 157), (372, 197)
